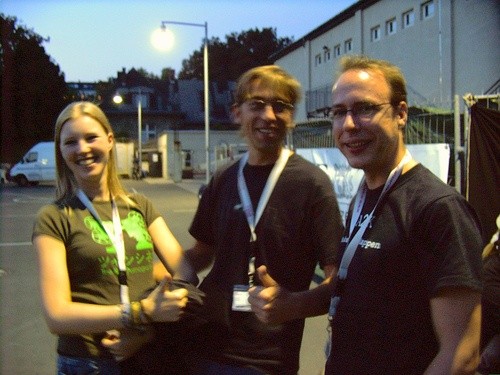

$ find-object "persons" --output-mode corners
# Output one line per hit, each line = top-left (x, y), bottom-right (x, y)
(474, 214), (500, 375)
(30, 99), (200, 375)
(150, 66), (344, 375)
(323, 52), (484, 375)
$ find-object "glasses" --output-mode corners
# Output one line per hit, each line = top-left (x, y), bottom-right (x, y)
(325, 103), (392, 122)
(244, 100), (293, 114)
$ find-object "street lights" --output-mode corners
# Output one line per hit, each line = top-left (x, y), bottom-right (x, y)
(113, 90), (143, 178)
(160, 20), (211, 186)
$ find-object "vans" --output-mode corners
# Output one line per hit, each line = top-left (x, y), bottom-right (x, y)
(8, 141), (57, 188)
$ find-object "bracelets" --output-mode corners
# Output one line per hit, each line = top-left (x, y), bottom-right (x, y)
(117, 301), (135, 332)
(134, 293), (153, 333)
